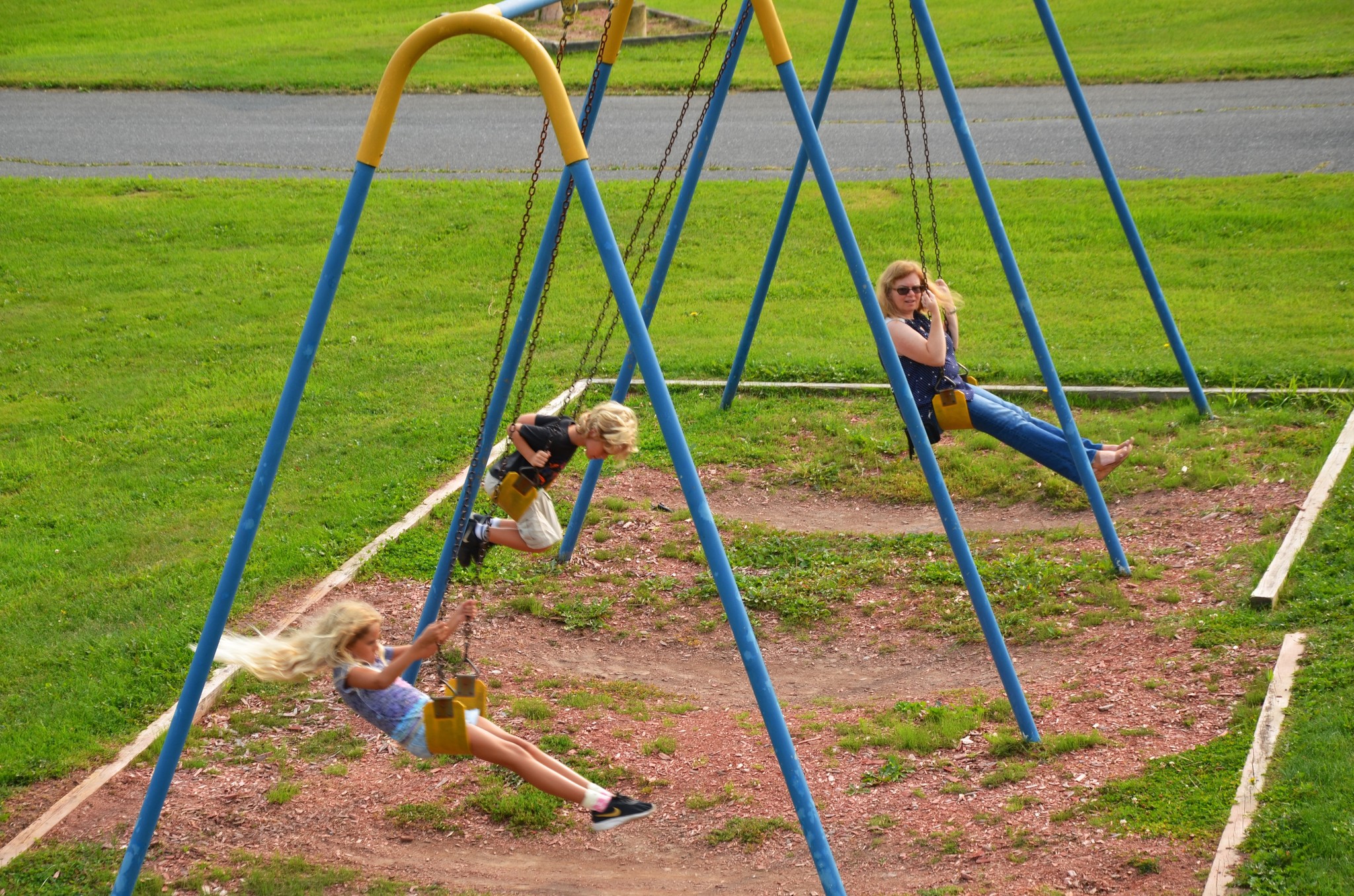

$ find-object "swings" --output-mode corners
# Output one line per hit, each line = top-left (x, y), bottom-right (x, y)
(491, 0), (753, 525)
(889, 0), (976, 429)
(422, 0), (617, 751)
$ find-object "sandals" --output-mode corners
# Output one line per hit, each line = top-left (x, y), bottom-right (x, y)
(1119, 437), (1134, 451)
(1093, 444), (1133, 482)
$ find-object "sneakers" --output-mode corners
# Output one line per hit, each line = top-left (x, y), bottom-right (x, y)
(471, 513), (494, 566)
(615, 794), (656, 809)
(457, 519), (478, 567)
(590, 798), (655, 831)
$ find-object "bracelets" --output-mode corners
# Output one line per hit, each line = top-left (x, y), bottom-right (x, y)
(945, 307), (956, 316)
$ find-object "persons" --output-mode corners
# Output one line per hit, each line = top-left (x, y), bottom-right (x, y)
(874, 261), (1135, 487)
(188, 599), (656, 831)
(457, 400), (639, 568)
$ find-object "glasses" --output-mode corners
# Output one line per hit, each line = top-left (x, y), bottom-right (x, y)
(893, 285), (924, 296)
